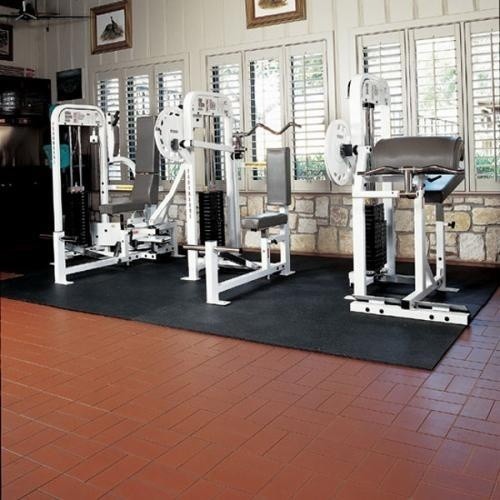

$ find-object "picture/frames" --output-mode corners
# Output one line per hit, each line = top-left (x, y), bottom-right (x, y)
(244, 0), (306, 28)
(89, 0), (132, 55)
(0, 23), (13, 61)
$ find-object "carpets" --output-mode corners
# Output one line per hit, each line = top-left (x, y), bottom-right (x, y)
(0, 245), (500, 370)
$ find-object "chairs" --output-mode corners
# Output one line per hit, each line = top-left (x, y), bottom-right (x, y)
(99, 114), (159, 215)
(240, 147), (291, 230)
